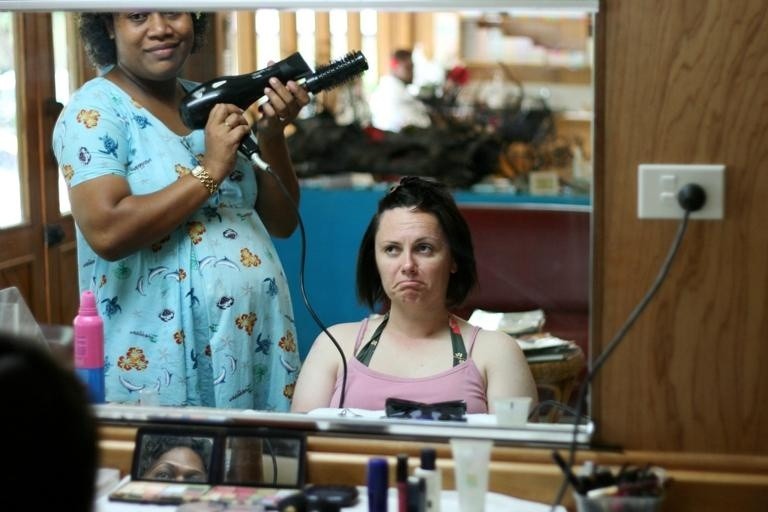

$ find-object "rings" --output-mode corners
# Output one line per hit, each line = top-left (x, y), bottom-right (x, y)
(279, 116), (290, 122)
(224, 120), (233, 128)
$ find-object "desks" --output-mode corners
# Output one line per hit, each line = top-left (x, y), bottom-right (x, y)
(517, 333), (589, 424)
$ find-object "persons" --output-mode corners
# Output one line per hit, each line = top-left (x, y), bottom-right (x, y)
(45, 13), (309, 413)
(139, 437), (210, 485)
(370, 49), (431, 131)
(284, 177), (541, 424)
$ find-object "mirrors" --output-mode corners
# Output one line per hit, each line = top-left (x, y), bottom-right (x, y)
(133, 427), (309, 488)
(3, 1), (603, 453)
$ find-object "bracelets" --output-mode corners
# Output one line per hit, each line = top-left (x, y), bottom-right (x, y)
(190, 165), (219, 195)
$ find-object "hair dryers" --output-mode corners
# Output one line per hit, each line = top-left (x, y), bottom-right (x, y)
(179, 51), (315, 157)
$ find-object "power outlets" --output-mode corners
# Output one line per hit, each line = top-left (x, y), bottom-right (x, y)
(637, 162), (727, 222)
